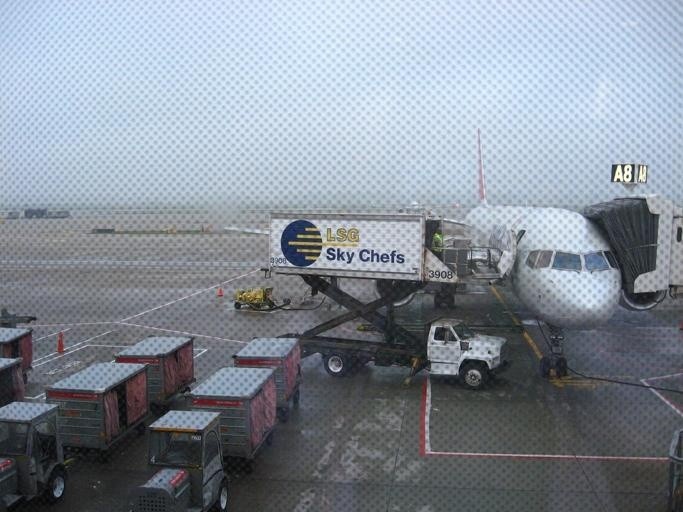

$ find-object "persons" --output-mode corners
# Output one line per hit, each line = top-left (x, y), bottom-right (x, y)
(429, 230), (443, 262)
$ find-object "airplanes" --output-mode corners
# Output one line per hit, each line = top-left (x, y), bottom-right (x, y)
(224, 126), (683, 381)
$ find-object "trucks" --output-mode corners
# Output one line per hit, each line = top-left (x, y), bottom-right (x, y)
(1, 336), (197, 512)
(126, 338), (303, 511)
(252, 208), (515, 391)
